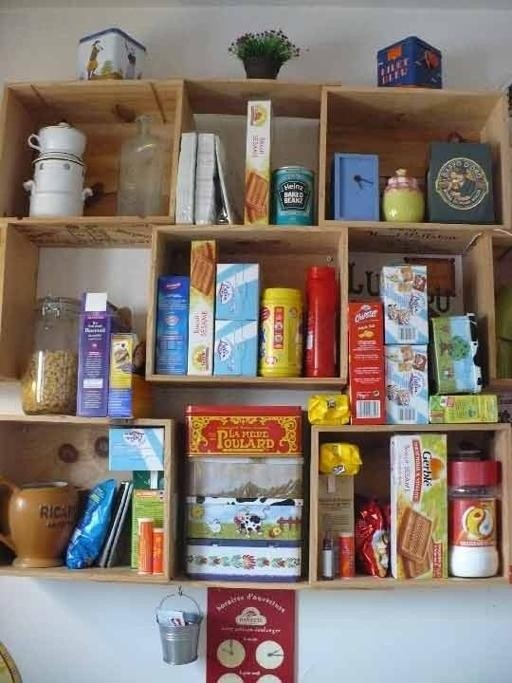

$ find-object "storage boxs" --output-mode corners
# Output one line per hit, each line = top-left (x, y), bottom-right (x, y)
(184, 406), (304, 583)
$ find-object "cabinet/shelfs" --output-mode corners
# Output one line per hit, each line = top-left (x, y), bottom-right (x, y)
(0, 78), (512, 590)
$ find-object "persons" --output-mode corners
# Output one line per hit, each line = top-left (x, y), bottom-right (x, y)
(87, 41), (104, 78)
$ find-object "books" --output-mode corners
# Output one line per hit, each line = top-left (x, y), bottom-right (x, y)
(98, 481), (134, 570)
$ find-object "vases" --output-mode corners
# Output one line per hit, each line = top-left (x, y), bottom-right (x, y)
(242, 55), (286, 80)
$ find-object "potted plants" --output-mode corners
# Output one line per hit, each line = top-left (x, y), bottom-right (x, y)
(225, 27), (309, 63)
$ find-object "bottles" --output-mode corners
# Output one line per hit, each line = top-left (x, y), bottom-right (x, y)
(117, 112), (169, 219)
(319, 538), (337, 581)
(304, 264), (341, 378)
(16, 293), (82, 415)
(22, 152), (93, 220)
(381, 166), (428, 224)
(258, 286), (305, 380)
(448, 456), (503, 579)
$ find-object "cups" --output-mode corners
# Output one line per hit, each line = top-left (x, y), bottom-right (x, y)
(27, 120), (90, 157)
(0, 473), (76, 569)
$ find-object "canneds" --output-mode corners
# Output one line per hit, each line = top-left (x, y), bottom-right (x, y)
(272, 166), (315, 226)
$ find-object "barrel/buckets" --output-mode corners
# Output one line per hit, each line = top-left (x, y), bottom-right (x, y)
(155, 593), (204, 666)
